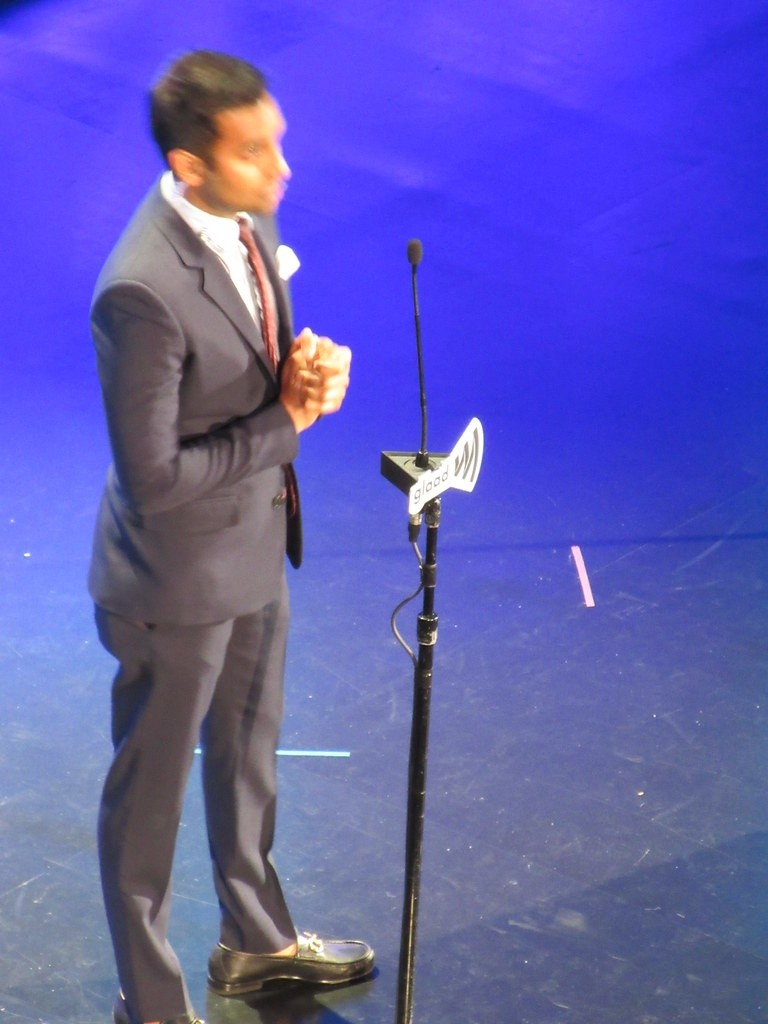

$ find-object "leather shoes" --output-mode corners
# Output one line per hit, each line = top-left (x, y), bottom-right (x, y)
(206, 925), (376, 997)
(114, 983), (207, 1023)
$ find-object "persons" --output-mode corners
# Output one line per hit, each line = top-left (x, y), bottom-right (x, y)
(86, 50), (377, 1024)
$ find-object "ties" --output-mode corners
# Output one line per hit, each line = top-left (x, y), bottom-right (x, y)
(237, 218), (296, 517)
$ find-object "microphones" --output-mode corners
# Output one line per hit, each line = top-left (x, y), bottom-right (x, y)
(406, 238), (428, 467)
